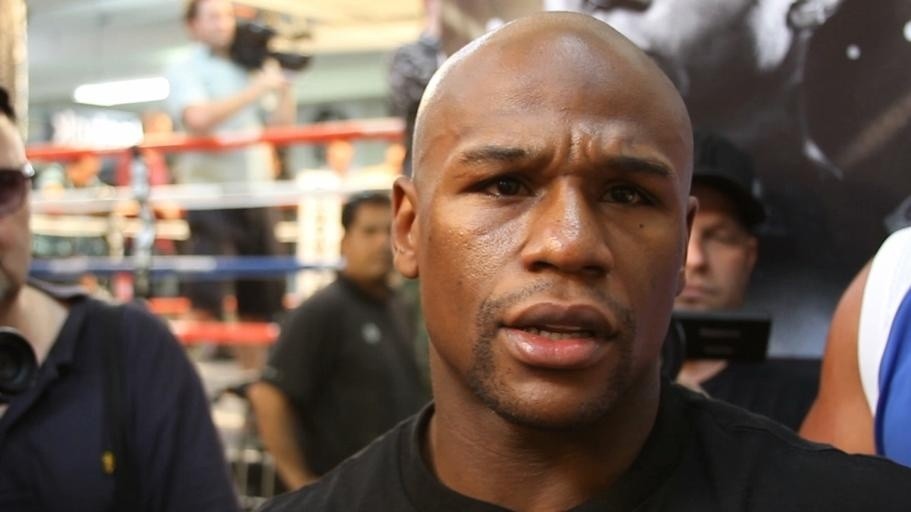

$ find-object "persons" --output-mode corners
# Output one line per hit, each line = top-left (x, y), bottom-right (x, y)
(247, 184), (435, 495)
(149, 0), (289, 401)
(110, 104), (182, 300)
(24, 141), (108, 289)
(660, 126), (822, 434)
(789, 222), (911, 474)
(292, 99), (401, 309)
(378, 0), (505, 185)
(0, 83), (246, 512)
(248, 9), (909, 510)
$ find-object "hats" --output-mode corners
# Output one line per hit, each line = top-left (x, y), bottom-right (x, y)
(689, 139), (767, 222)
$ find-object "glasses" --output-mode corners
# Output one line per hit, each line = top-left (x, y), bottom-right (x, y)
(2, 162), (36, 215)
